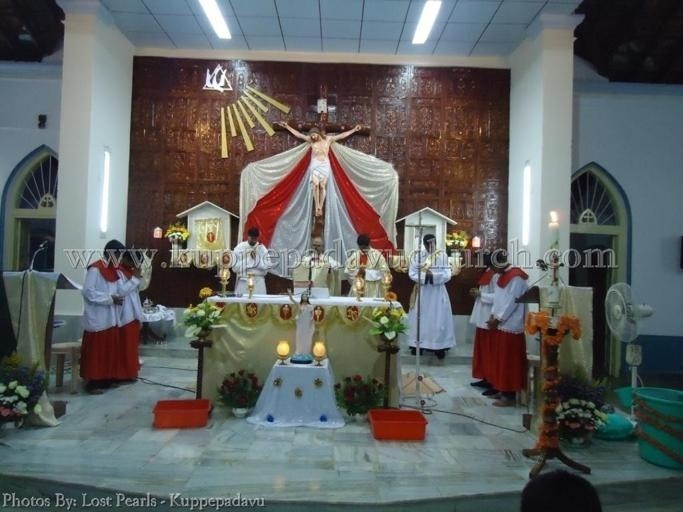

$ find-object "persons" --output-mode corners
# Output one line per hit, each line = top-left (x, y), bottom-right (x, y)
(300, 237), (341, 297)
(518, 469), (601, 512)
(469, 252), (500, 391)
(485, 253), (528, 407)
(407, 233), (456, 360)
(273, 120), (363, 217)
(116, 249), (142, 387)
(79, 240), (124, 395)
(230, 228), (270, 294)
(343, 234), (389, 298)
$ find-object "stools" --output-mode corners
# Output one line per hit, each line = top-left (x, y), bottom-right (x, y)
(48, 342), (84, 394)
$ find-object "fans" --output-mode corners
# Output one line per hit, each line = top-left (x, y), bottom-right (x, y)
(604, 281), (653, 428)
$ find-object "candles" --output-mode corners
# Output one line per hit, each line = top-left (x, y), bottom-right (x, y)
(153, 226), (162, 238)
(249, 277), (252, 285)
(547, 211), (558, 245)
(471, 236), (479, 247)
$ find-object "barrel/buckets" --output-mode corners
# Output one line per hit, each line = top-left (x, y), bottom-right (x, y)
(632, 387), (682, 469)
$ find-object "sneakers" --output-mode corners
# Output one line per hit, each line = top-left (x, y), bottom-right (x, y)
(411, 346), (447, 361)
(84, 379), (123, 395)
(470, 377), (516, 409)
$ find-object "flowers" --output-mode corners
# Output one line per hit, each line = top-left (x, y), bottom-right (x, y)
(163, 222), (189, 243)
(361, 291), (410, 341)
(215, 369), (263, 409)
(333, 374), (388, 418)
(557, 363), (613, 440)
(445, 230), (469, 251)
(0, 350), (47, 417)
(178, 286), (223, 336)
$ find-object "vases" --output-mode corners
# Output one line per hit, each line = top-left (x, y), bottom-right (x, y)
(351, 412), (368, 423)
(192, 324), (214, 342)
(231, 407), (250, 419)
(560, 427), (593, 448)
(376, 333), (397, 347)
